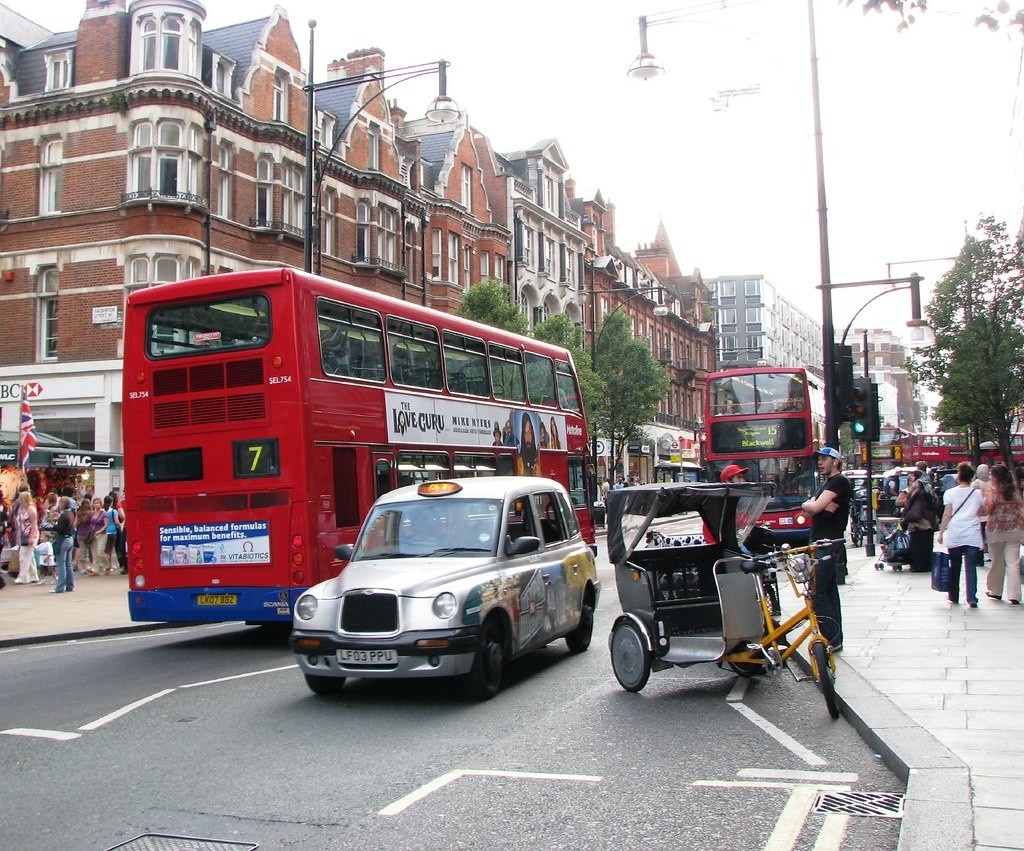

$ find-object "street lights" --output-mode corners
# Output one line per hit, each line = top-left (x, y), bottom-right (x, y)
(584, 259), (678, 531)
(714, 337), (769, 416)
(300, 14), (464, 279)
(625, 0), (929, 453)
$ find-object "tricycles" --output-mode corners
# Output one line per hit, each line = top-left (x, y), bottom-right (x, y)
(604, 478), (846, 721)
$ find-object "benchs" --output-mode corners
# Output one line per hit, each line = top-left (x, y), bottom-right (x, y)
(321, 328), (505, 399)
(629, 542), (721, 628)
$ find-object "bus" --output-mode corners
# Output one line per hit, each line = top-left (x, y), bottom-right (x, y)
(120, 265), (605, 639)
(845, 424), (1024, 546)
(704, 364), (842, 550)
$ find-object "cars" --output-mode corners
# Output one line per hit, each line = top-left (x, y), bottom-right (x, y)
(288, 475), (602, 700)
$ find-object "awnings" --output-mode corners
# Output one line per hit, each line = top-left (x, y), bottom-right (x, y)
(0, 444), (124, 470)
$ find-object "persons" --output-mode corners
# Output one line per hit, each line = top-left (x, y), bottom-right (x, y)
(884, 460), (1024, 608)
(703, 465), (760, 557)
(518, 412), (540, 477)
(536, 416), (561, 454)
(601, 474), (655, 514)
(800, 448), (853, 656)
(492, 412), (520, 455)
(0, 485), (127, 593)
(713, 398), (803, 416)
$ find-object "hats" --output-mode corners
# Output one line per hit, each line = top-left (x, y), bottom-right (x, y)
(720, 465), (749, 483)
(812, 447), (842, 463)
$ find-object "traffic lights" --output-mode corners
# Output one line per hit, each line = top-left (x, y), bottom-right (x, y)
(849, 377), (872, 441)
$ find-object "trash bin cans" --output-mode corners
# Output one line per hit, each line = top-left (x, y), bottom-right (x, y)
(594, 507), (606, 529)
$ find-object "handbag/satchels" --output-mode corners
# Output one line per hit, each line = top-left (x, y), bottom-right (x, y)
(930, 542), (950, 592)
(886, 530), (912, 564)
(85, 531), (93, 544)
(21, 532), (29, 545)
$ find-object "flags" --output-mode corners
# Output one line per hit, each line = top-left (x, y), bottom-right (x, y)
(21, 392), (37, 471)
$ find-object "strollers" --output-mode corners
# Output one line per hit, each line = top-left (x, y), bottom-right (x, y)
(875, 518), (906, 572)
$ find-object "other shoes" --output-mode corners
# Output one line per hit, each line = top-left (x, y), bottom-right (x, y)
(0, 558), (128, 592)
(1011, 599), (1020, 604)
(985, 591), (1002, 600)
(970, 601), (977, 607)
(946, 595), (958, 603)
(832, 643), (843, 652)
